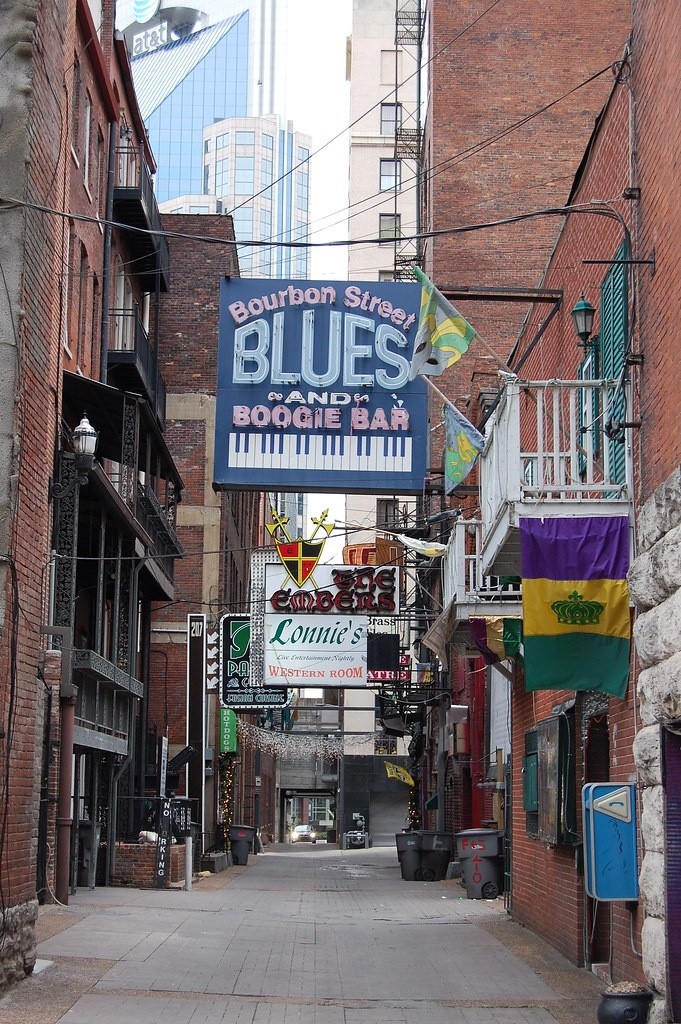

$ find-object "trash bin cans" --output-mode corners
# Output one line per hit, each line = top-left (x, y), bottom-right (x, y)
(418, 830), (450, 880)
(455, 828), (505, 899)
(229, 824), (254, 866)
(395, 832), (422, 881)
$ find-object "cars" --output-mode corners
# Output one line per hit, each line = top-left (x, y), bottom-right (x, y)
(292, 824), (316, 844)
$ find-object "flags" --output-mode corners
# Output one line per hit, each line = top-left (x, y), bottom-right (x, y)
(438, 400), (485, 495)
(407, 264), (477, 381)
(468, 614), (522, 665)
(518, 516), (632, 700)
(383, 760), (415, 788)
(416, 661), (433, 683)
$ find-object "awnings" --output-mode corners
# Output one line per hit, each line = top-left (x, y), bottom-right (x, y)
(63, 369), (185, 491)
